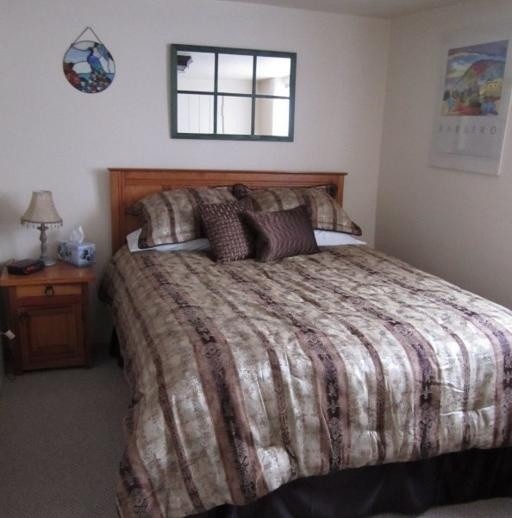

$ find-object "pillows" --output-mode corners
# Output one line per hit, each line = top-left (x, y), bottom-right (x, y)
(233, 184), (363, 236)
(197, 196), (256, 264)
(125, 185), (239, 249)
(242, 204), (322, 262)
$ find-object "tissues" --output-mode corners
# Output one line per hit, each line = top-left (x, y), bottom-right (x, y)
(56, 224), (97, 267)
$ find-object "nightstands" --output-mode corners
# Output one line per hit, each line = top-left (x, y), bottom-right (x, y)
(0, 259), (97, 374)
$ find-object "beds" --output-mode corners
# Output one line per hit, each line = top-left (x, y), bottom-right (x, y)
(98, 167), (512, 518)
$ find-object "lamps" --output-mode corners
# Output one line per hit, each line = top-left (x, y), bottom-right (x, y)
(20, 191), (63, 267)
